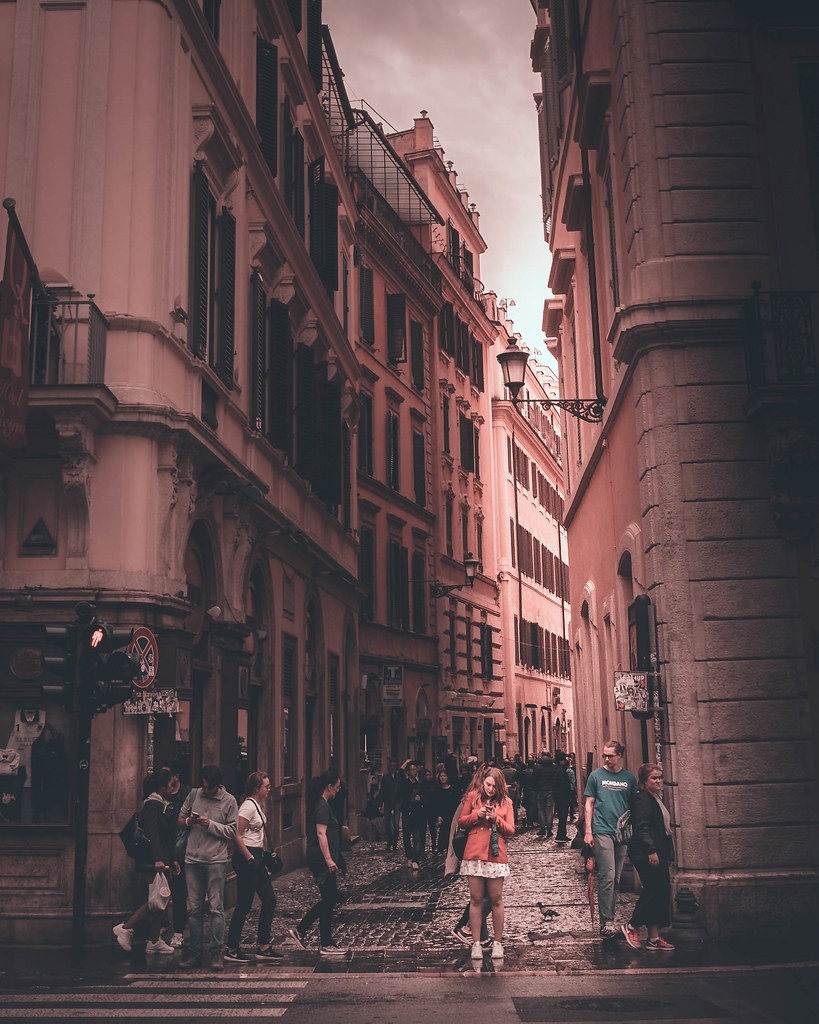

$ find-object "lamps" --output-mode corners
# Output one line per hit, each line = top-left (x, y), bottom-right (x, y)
(206, 603), (222, 618)
(430, 552), (479, 600)
(497, 336), (603, 424)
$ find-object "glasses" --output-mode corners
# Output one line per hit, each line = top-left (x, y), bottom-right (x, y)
(602, 754), (619, 759)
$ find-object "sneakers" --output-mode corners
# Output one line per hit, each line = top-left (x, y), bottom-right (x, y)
(480, 938), (494, 951)
(646, 937), (675, 950)
(113, 920), (349, 970)
(471, 941), (483, 959)
(600, 923), (616, 939)
(492, 940), (504, 958)
(449, 927), (473, 946)
(621, 922), (642, 949)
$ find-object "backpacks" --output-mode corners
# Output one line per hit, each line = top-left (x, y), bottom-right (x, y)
(616, 810), (634, 846)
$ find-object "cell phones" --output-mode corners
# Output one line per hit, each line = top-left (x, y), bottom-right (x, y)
(192, 812), (199, 818)
(486, 806), (493, 812)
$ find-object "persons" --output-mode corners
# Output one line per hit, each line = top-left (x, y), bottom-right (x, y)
(177, 765), (239, 971)
(493, 749), (578, 842)
(583, 741), (637, 939)
(288, 770), (347, 955)
(224, 772), (284, 963)
(444, 761), (515, 959)
(159, 763), (192, 949)
(376, 757), (461, 872)
(112, 769), (175, 955)
(620, 762), (675, 950)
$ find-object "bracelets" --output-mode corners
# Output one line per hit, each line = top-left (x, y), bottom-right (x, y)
(185, 817), (191, 826)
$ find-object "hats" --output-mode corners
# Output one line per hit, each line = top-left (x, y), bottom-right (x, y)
(555, 752), (569, 761)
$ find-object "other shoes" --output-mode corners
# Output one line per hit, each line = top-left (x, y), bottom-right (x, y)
(547, 832), (553, 838)
(554, 836), (572, 842)
(407, 858), (419, 869)
(537, 830), (546, 835)
(570, 816), (576, 821)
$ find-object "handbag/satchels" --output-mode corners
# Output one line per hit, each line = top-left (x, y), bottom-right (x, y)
(263, 850), (283, 873)
(341, 832), (352, 851)
(452, 825), (470, 861)
(175, 788), (197, 847)
(118, 813), (153, 858)
(148, 871), (171, 911)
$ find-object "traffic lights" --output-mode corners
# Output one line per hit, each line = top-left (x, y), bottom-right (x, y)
(79, 624), (112, 713)
(41, 625), (77, 710)
(111, 625), (136, 708)
